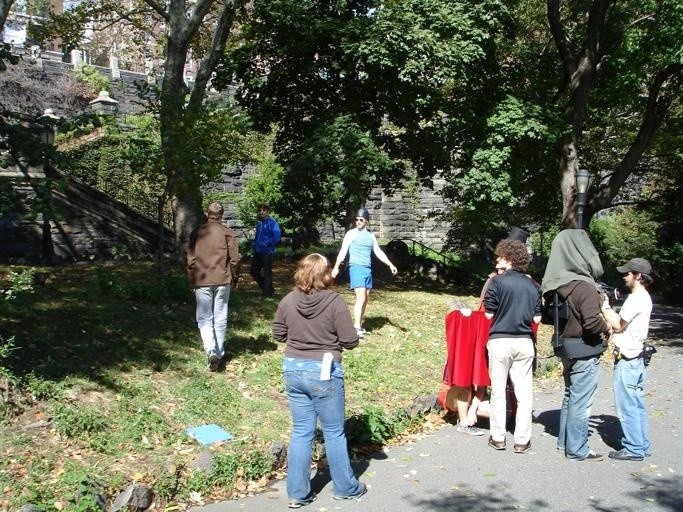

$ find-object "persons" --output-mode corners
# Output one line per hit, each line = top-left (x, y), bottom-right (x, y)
(272, 253), (367, 508)
(438, 228), (543, 453)
(600, 258), (653, 461)
(186, 203), (239, 372)
(331, 208), (398, 335)
(250, 204), (281, 296)
(540, 229), (615, 461)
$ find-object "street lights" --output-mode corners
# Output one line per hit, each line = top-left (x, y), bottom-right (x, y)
(570, 167), (593, 231)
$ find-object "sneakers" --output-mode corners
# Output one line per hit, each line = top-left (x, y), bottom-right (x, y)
(332, 483), (367, 500)
(207, 350), (219, 371)
(565, 451), (605, 461)
(356, 327), (367, 336)
(513, 441), (532, 453)
(488, 435), (506, 450)
(287, 491), (318, 509)
(557, 445), (565, 450)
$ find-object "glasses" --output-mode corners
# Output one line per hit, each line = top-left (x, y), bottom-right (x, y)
(355, 219), (364, 222)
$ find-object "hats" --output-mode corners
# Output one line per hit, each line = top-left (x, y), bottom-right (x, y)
(616, 257), (652, 274)
(354, 207), (370, 219)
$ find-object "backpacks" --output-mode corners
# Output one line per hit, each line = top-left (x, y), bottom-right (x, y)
(540, 290), (570, 325)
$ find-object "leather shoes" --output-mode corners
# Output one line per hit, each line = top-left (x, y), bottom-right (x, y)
(608, 446), (645, 461)
(644, 453), (652, 457)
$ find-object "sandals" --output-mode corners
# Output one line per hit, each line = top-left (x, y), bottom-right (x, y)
(457, 418), (484, 436)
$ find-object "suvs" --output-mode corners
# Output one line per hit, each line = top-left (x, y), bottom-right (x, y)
(598, 281), (614, 292)
